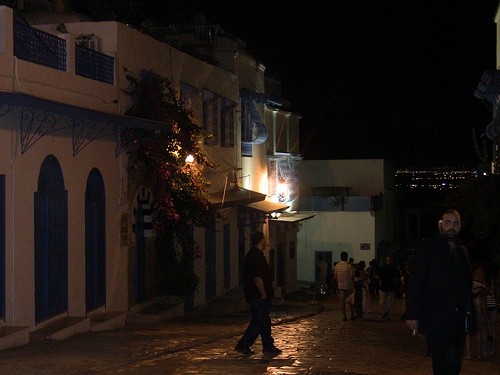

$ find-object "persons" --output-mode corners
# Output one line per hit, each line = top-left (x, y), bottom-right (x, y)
(401, 208), (479, 374)
(235, 231), (282, 355)
(326, 251), (413, 321)
(465, 264), (500, 360)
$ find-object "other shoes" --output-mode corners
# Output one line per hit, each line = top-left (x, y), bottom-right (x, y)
(343, 315), (364, 320)
(382, 310), (392, 320)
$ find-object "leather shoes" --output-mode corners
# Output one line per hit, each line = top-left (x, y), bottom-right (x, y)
(235, 342), (255, 355)
(262, 344), (282, 353)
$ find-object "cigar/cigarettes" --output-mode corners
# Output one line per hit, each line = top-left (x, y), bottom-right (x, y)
(412, 325), (416, 337)
